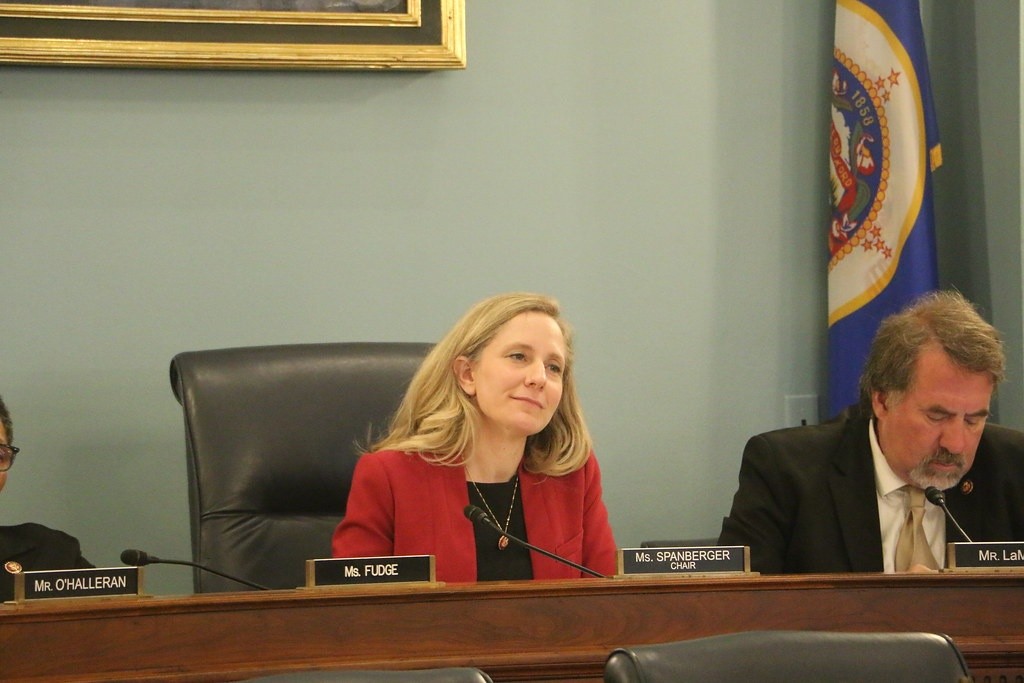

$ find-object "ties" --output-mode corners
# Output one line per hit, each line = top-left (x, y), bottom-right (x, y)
(895, 486), (944, 572)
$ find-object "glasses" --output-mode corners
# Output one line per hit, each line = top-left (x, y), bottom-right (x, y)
(0, 443), (19, 470)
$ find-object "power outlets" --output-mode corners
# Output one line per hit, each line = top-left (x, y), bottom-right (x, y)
(784, 392), (820, 430)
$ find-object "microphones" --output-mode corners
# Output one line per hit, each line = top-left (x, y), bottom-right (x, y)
(464, 504), (608, 577)
(121, 549), (271, 590)
(925, 486), (973, 542)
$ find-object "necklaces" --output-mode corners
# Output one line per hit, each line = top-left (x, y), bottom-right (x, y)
(455, 442), (524, 552)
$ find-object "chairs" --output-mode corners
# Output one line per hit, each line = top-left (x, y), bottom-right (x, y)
(602, 629), (973, 683)
(168, 341), (439, 598)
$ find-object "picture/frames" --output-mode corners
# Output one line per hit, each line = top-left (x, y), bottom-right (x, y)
(0, 0), (466, 72)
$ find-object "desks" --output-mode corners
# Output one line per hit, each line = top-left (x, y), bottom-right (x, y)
(1, 571), (1024, 683)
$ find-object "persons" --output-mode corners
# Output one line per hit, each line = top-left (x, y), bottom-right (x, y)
(1, 394), (96, 603)
(713, 289), (1023, 577)
(328, 296), (623, 585)
